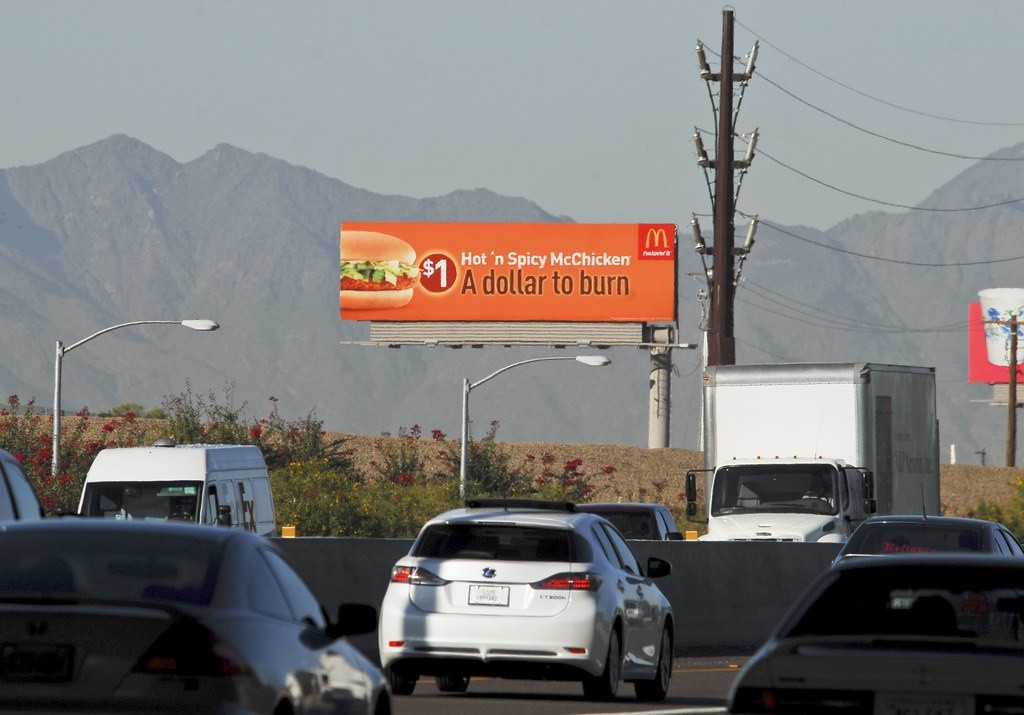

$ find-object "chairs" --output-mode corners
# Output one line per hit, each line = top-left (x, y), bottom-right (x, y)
(454, 536), (577, 565)
(169, 495), (199, 522)
(735, 481), (762, 509)
(95, 489), (126, 522)
(902, 596), (960, 636)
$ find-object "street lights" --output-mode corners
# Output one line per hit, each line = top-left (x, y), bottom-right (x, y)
(459, 354), (612, 504)
(50, 319), (221, 475)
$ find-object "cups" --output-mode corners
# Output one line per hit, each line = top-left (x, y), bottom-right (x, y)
(978, 288), (1024, 367)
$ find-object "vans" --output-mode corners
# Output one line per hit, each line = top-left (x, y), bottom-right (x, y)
(75, 436), (278, 540)
(571, 504), (685, 544)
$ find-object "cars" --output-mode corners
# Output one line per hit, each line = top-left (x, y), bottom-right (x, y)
(0, 449), (395, 715)
(725, 514), (1024, 715)
(377, 498), (682, 702)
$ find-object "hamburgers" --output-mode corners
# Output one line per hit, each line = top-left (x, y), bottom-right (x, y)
(339, 230), (417, 310)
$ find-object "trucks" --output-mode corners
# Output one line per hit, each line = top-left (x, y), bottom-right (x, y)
(684, 360), (943, 544)
